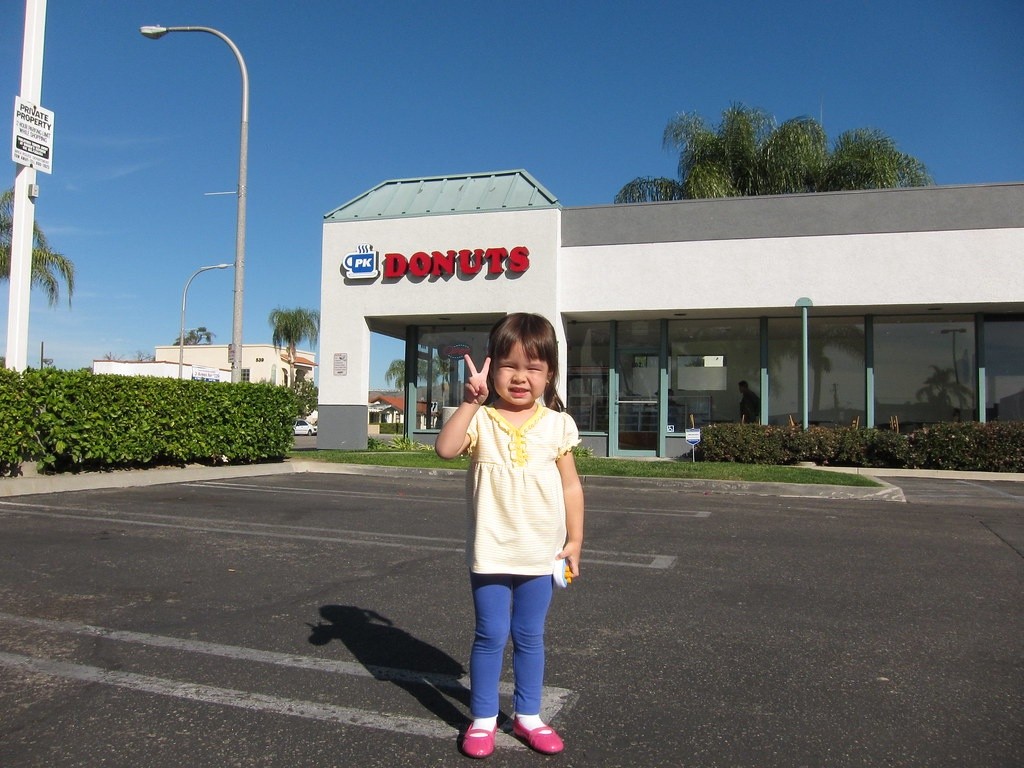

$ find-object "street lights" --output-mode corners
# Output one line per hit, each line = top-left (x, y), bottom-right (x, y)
(138, 24), (250, 383)
(940, 328), (968, 420)
(178, 264), (231, 381)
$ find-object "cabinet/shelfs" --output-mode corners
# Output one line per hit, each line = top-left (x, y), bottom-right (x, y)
(618, 395), (710, 434)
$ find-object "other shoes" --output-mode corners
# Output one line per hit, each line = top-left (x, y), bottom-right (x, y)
(462, 721), (498, 758)
(512, 710), (564, 754)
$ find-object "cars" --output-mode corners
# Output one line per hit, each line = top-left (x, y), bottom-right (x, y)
(291, 419), (318, 436)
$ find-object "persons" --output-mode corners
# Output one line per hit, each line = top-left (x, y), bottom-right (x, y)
(668, 389), (680, 424)
(738, 381), (760, 424)
(435, 313), (585, 758)
